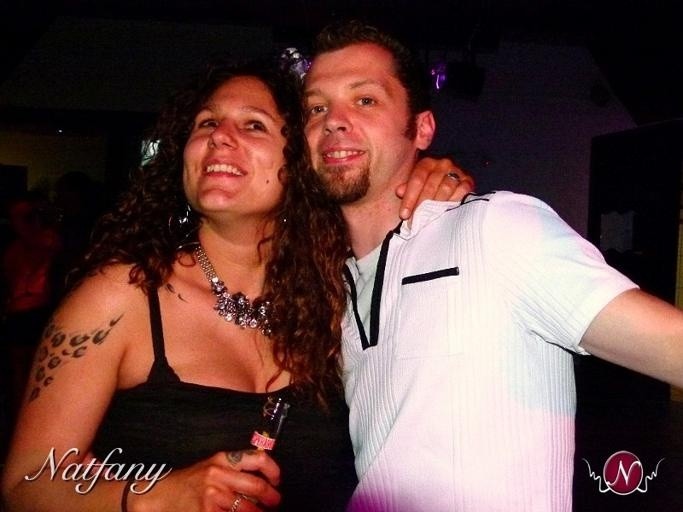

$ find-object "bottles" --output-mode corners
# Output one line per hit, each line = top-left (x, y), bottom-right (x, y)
(234, 395), (290, 506)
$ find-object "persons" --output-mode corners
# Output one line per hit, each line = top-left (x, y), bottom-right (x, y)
(0, 17), (683, 512)
